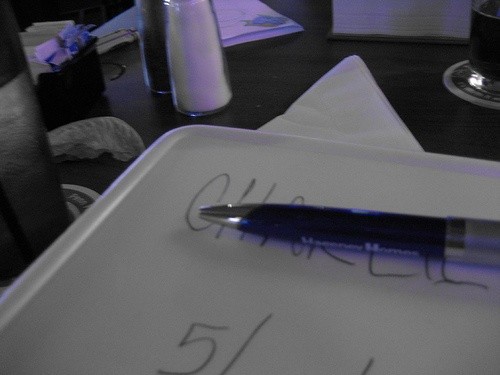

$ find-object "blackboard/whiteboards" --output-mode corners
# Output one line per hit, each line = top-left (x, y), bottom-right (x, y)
(0, 124), (498, 375)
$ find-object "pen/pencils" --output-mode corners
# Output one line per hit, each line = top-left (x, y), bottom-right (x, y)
(199, 203), (499, 270)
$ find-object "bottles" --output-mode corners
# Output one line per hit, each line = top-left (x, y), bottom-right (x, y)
(134, 0), (175, 93)
(1, 6), (69, 283)
(162, 1), (233, 118)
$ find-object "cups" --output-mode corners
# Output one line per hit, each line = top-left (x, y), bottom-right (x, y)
(466, 1), (500, 84)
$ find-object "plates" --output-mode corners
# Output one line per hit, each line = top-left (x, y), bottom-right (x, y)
(442, 60), (499, 113)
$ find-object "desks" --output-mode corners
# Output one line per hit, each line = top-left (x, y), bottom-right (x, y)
(36, 0), (500, 221)
